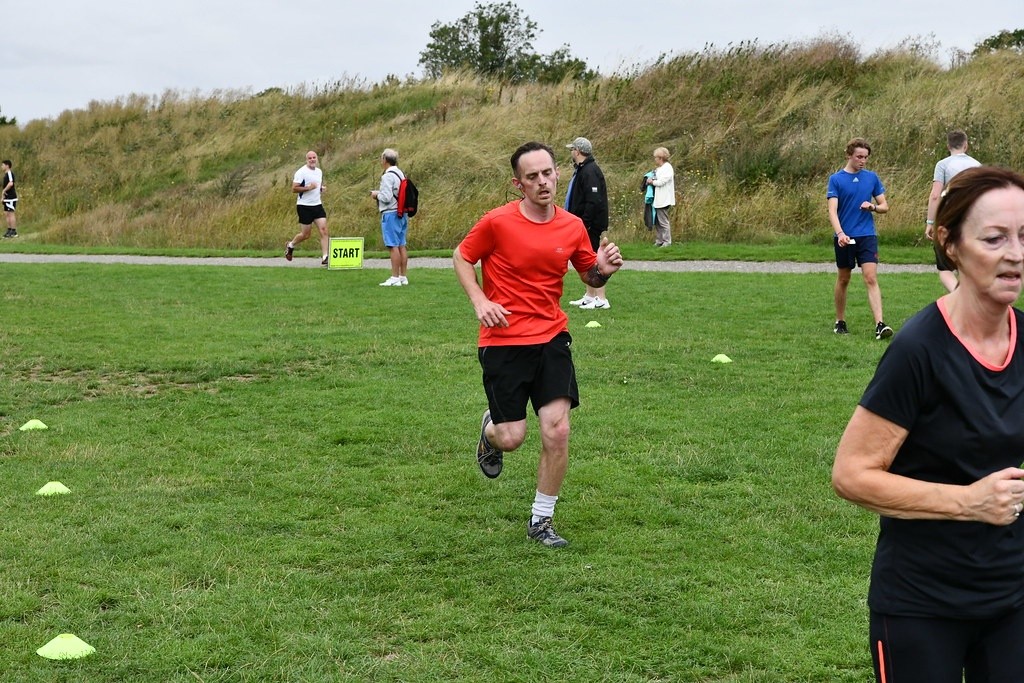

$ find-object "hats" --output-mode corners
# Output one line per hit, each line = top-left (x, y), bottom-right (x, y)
(566, 137), (592, 153)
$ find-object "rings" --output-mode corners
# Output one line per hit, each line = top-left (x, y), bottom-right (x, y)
(1014, 505), (1020, 517)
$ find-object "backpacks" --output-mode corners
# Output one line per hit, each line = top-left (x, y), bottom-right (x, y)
(388, 171), (418, 217)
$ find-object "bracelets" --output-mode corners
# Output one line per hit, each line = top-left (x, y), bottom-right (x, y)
(596, 265), (611, 281)
(925, 219), (934, 225)
(873, 205), (876, 211)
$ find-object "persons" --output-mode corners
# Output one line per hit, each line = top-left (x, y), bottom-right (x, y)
(827, 138), (893, 340)
(452, 142), (623, 546)
(566, 137), (611, 309)
(285, 150), (328, 264)
(925, 129), (982, 293)
(1, 160), (18, 239)
(646, 147), (676, 247)
(831, 167), (1024, 683)
(370, 148), (408, 286)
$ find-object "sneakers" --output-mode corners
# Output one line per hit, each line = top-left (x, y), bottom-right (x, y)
(476, 410), (503, 478)
(834, 320), (850, 335)
(379, 279), (401, 287)
(399, 276), (408, 285)
(875, 322), (894, 340)
(526, 516), (568, 549)
(579, 296), (610, 309)
(569, 294), (594, 305)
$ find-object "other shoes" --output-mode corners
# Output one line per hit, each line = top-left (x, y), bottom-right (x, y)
(3, 230), (18, 239)
(321, 256), (328, 266)
(285, 241), (294, 261)
